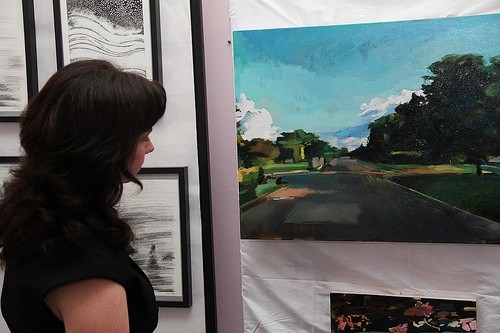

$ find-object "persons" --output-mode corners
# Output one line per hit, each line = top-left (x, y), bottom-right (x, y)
(0, 57), (167, 333)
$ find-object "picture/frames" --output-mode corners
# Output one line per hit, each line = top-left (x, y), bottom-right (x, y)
(53, 0), (163, 90)
(0, 155), (30, 254)
(0, 0), (40, 123)
(112, 166), (192, 309)
